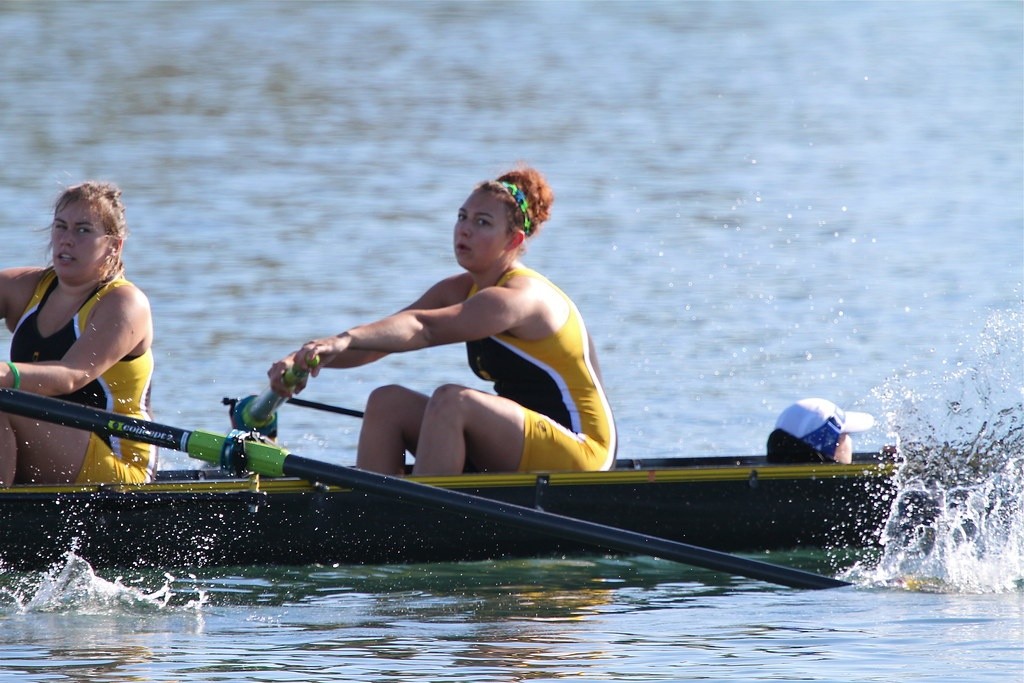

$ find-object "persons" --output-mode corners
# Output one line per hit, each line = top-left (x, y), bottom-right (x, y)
(767, 399), (878, 466)
(0, 179), (160, 484)
(268, 165), (620, 476)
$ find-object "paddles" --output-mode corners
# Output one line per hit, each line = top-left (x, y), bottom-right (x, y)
(220, 352), (322, 441)
(0, 389), (857, 591)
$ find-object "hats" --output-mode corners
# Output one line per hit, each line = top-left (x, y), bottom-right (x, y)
(776, 398), (874, 459)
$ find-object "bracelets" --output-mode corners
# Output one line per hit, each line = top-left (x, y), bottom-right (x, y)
(7, 358), (20, 391)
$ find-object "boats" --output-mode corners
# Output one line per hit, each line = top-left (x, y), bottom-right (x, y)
(0, 453), (1007, 566)
